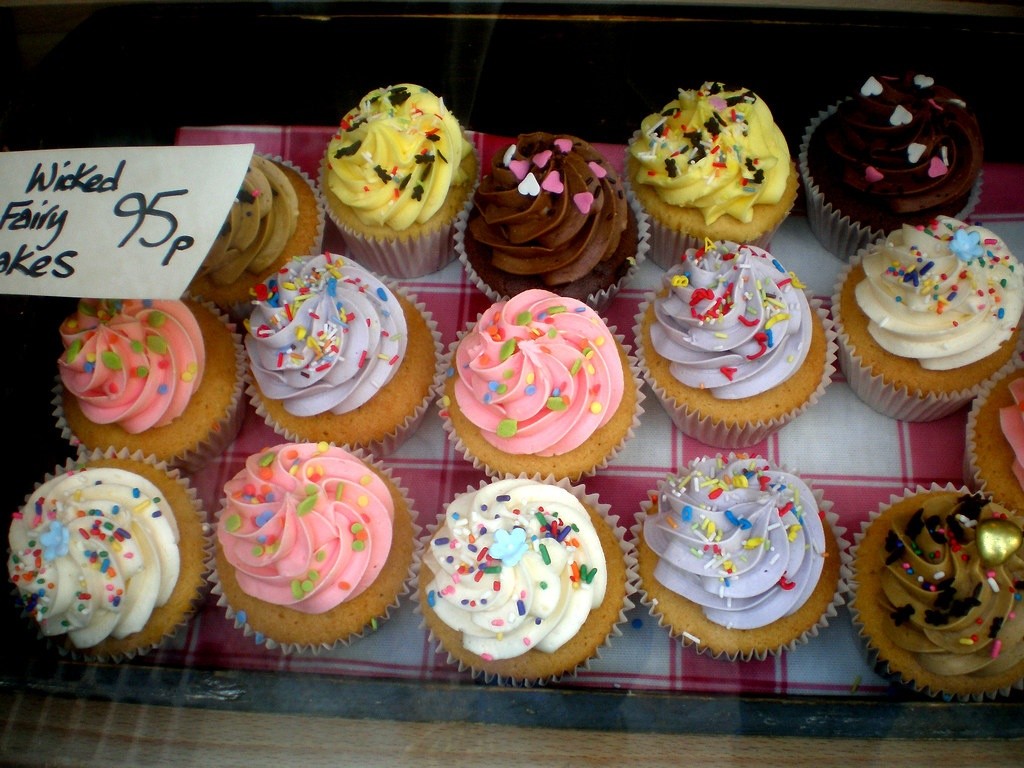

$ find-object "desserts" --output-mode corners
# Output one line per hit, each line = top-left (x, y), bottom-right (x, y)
(7, 70), (1024, 705)
